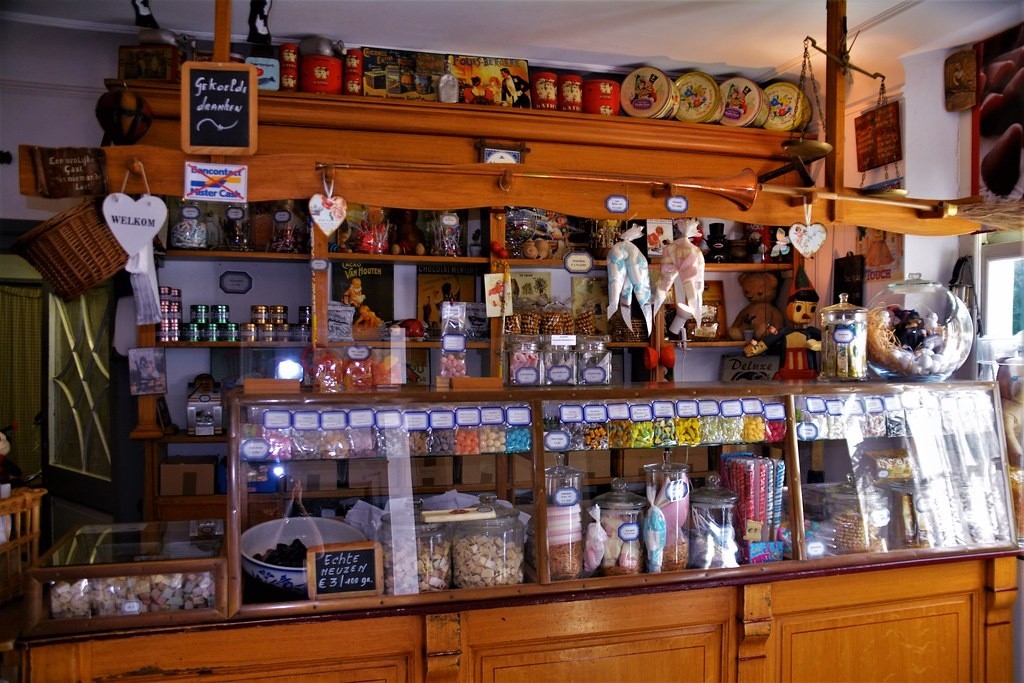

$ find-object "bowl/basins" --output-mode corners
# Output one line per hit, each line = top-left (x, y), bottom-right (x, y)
(240, 517), (368, 590)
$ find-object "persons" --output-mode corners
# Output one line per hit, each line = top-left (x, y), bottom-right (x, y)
(744, 280), (822, 383)
(136, 356), (160, 393)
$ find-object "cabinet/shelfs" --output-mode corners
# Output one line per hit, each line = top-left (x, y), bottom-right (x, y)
(105, 127), (804, 529)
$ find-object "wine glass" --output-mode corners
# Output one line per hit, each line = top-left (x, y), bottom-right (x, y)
(503, 210), (537, 259)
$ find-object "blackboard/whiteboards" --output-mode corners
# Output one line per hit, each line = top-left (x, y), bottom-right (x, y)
(182, 62), (258, 156)
(305, 540), (382, 598)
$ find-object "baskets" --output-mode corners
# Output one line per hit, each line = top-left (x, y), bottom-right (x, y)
(17, 199), (129, 303)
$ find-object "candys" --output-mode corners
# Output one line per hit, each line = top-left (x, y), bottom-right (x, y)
(544, 415), (788, 449)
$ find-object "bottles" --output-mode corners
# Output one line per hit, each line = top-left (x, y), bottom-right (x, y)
(508, 342), (613, 386)
(167, 194), (466, 257)
(157, 285), (312, 343)
(296, 37), (348, 58)
(867, 273), (974, 382)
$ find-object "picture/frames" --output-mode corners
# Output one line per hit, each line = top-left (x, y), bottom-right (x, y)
(118, 45), (178, 83)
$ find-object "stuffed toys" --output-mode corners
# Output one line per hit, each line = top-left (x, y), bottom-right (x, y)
(727, 272), (783, 341)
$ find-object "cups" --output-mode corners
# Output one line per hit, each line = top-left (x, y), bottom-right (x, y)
(593, 477), (646, 577)
(451, 492), (525, 587)
(825, 472), (889, 555)
(816, 293), (869, 382)
(379, 499), (451, 595)
(689, 472), (740, 568)
(543, 453), (584, 580)
(642, 451), (691, 573)
